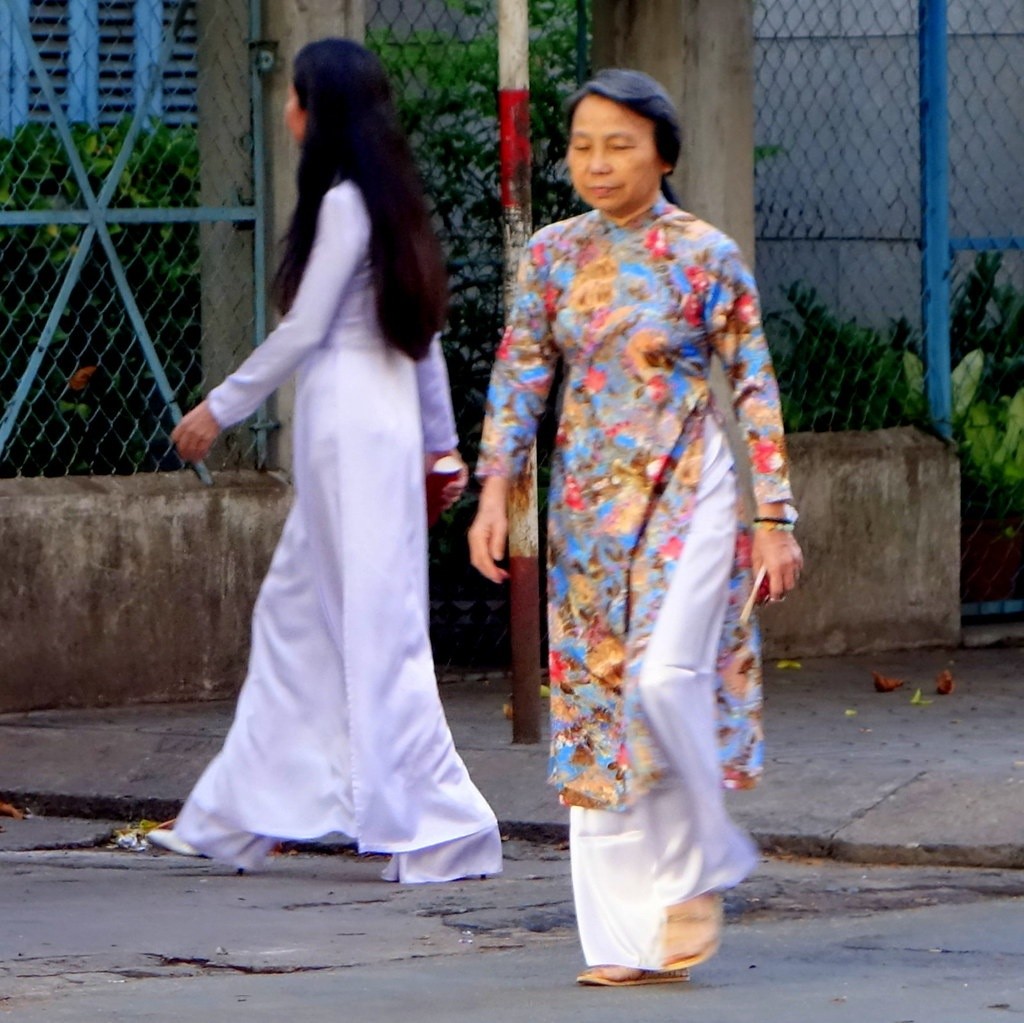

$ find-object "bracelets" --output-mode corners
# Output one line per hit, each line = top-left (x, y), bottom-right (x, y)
(750, 515), (796, 532)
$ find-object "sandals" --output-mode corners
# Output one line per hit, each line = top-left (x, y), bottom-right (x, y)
(662, 891), (724, 970)
(577, 964), (690, 986)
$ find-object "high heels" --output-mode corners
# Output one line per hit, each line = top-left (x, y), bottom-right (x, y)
(145, 829), (244, 876)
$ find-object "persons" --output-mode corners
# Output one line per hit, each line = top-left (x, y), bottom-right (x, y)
(146, 38), (505, 884)
(467, 67), (805, 988)
